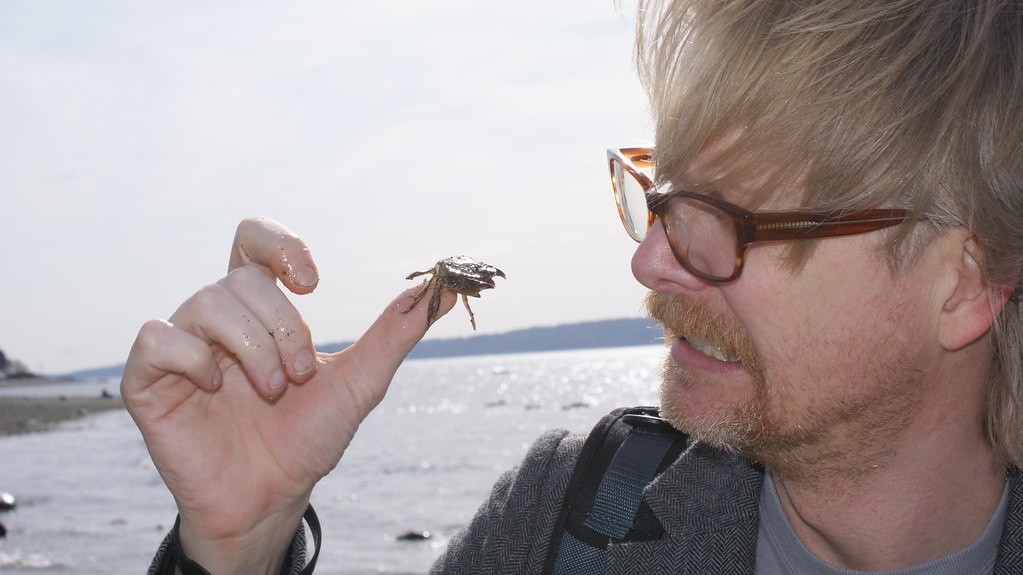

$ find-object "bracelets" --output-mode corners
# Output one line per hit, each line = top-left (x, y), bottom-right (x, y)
(173, 503), (323, 575)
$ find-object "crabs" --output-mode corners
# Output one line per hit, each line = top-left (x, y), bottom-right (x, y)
(399, 254), (507, 332)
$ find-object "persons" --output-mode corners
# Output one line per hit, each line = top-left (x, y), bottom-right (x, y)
(117, 0), (1023, 575)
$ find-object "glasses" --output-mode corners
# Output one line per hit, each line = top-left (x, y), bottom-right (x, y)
(606, 146), (927, 283)
